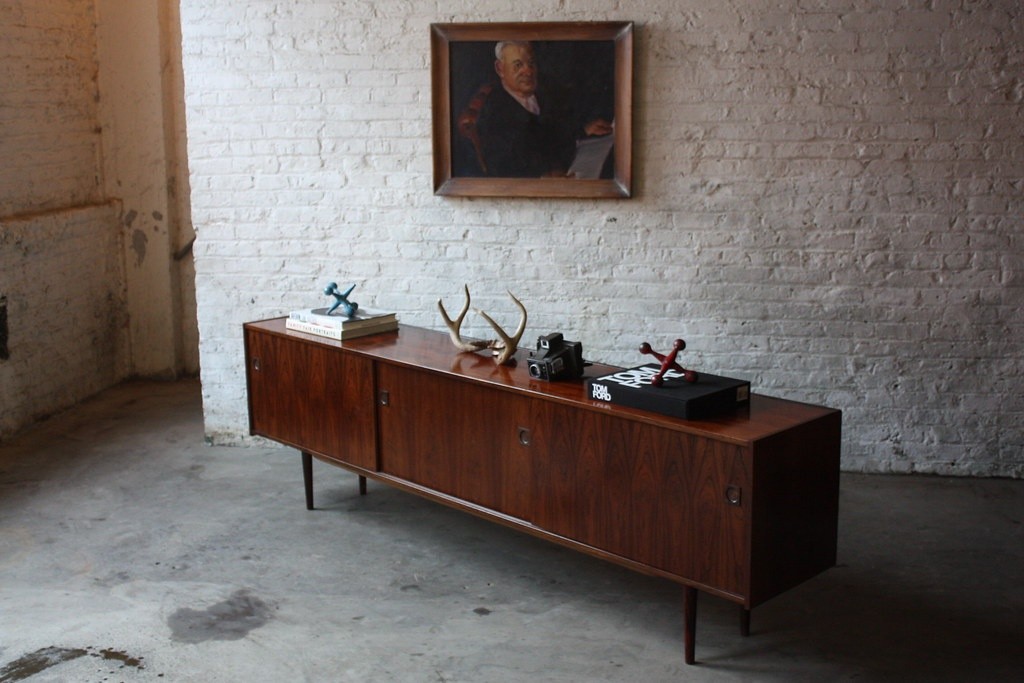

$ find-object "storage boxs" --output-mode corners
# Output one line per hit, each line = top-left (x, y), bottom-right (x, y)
(587, 364), (751, 420)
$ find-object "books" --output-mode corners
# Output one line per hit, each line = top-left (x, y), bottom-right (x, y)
(289, 304), (397, 329)
(284, 317), (400, 341)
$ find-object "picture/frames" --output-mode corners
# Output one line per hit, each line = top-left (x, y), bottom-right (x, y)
(431, 21), (633, 199)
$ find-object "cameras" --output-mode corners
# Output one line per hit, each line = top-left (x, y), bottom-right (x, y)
(526, 332), (585, 383)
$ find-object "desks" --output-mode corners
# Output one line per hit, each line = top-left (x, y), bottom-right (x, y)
(242, 306), (842, 662)
